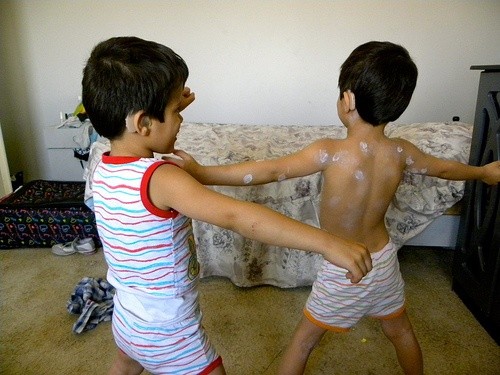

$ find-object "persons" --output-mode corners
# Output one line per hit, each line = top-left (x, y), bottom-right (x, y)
(82, 37), (371, 374)
(163, 42), (499, 374)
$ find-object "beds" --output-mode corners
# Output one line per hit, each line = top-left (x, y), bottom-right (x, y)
(97, 121), (474, 289)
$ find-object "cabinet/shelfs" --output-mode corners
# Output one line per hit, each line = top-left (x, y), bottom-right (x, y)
(448, 65), (500, 349)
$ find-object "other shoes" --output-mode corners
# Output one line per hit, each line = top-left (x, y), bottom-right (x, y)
(52, 237), (97, 257)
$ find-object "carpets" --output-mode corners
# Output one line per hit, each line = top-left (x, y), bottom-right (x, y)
(0, 247), (500, 375)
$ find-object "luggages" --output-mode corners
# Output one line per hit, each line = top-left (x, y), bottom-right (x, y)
(0, 178), (103, 249)
